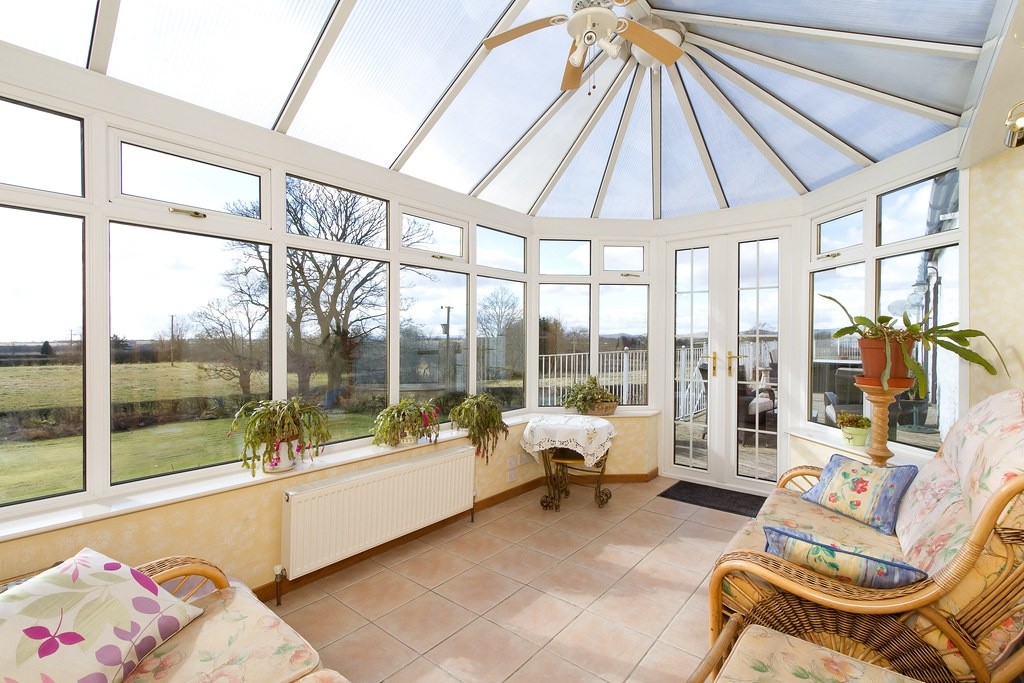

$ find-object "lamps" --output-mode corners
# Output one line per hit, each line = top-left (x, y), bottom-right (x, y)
(570, 34), (589, 68)
(582, 15), (597, 45)
(598, 27), (622, 61)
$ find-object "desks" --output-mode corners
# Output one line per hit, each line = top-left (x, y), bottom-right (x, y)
(521, 414), (613, 511)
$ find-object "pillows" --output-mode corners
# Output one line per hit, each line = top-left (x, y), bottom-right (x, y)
(0, 547), (204, 682)
(762, 525), (929, 588)
(802, 454), (919, 535)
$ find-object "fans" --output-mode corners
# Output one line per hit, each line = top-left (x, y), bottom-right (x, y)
(481, 0), (685, 91)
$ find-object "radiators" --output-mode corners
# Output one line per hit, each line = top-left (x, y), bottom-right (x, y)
(275, 443), (477, 607)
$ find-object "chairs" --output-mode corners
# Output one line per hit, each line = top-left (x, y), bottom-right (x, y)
(824, 368), (897, 440)
(683, 614), (930, 682)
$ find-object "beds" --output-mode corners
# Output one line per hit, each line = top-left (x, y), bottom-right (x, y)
(0, 555), (354, 683)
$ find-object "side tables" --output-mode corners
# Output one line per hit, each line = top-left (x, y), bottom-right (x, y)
(766, 407), (818, 449)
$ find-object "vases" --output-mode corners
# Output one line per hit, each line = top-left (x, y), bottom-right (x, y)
(257, 432), (299, 472)
(395, 423), (418, 446)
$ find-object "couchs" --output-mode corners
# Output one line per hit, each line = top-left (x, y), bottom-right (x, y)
(699, 364), (775, 448)
(708, 389), (1024, 683)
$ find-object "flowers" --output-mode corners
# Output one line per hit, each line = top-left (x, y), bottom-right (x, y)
(369, 399), (443, 445)
(226, 398), (331, 477)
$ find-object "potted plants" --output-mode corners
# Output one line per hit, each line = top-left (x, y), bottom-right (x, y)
(448, 392), (509, 465)
(895, 356), (930, 426)
(562, 377), (620, 415)
(836, 408), (872, 445)
(817, 293), (1011, 387)
